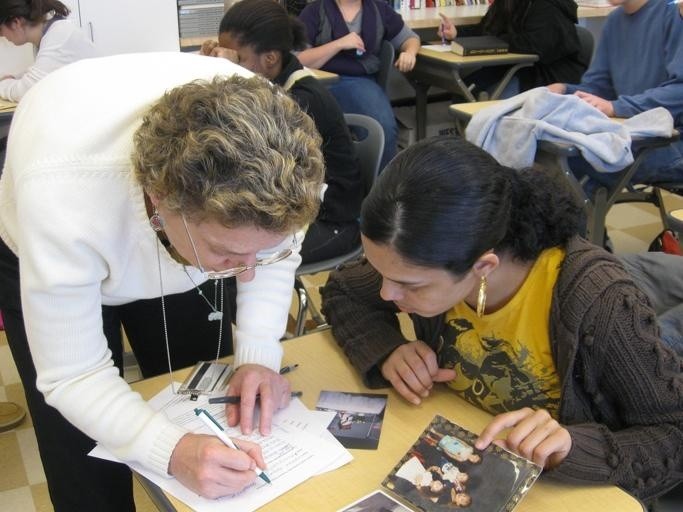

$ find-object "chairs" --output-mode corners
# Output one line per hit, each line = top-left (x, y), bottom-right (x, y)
(377, 39), (395, 90)
(574, 26), (594, 74)
(295, 113), (384, 336)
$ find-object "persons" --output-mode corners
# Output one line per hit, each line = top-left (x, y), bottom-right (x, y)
(0, 53), (327, 511)
(435, 0), (585, 105)
(289, 0), (420, 177)
(394, 428), (483, 508)
(541, 1), (682, 252)
(0, 0), (105, 101)
(199, 1), (366, 266)
(320, 135), (683, 511)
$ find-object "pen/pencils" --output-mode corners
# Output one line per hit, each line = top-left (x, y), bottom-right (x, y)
(279, 363), (299, 374)
(209, 391), (302, 404)
(194, 408), (273, 487)
(440, 17), (446, 49)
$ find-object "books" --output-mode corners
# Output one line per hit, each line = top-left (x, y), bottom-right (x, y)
(449, 34), (514, 58)
(384, 0), (494, 10)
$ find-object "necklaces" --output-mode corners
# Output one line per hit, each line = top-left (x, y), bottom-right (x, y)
(149, 211), (224, 322)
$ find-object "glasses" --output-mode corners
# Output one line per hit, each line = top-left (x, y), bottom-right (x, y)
(181, 215), (297, 279)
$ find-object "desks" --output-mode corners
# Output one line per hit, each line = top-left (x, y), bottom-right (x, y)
(447, 102), (680, 249)
(397, 46), (541, 140)
(128, 328), (646, 511)
(666, 208), (683, 231)
(1, 99), (16, 124)
(310, 68), (341, 85)
